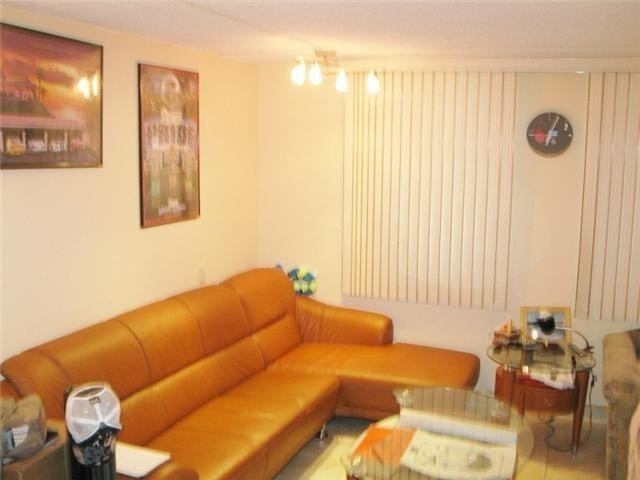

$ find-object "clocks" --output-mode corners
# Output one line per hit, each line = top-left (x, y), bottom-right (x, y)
(526, 111), (573, 159)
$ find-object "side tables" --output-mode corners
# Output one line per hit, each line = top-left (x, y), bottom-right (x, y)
(484, 341), (595, 455)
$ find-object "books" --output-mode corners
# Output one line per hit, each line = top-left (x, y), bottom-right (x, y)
(398, 427), (518, 480)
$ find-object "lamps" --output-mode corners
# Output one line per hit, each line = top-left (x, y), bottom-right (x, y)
(536, 309), (596, 357)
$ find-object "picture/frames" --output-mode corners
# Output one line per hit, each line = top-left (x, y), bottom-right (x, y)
(520, 306), (572, 347)
(138, 63), (200, 229)
(0, 22), (104, 170)
